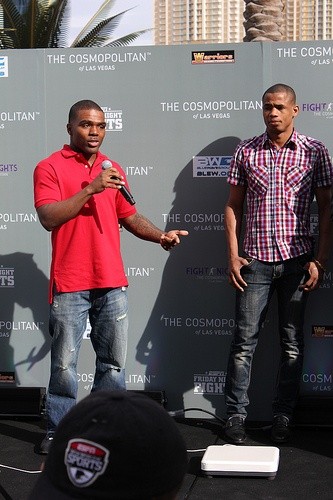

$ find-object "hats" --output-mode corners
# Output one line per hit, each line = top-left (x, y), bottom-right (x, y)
(30, 390), (185, 500)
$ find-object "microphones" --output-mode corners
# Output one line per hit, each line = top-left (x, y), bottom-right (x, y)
(102, 160), (136, 205)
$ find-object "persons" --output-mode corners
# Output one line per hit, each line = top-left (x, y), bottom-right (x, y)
(33, 99), (189, 454)
(28, 388), (191, 500)
(223, 84), (333, 443)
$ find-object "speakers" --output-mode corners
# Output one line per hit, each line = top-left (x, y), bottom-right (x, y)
(0, 386), (47, 419)
(128, 390), (168, 410)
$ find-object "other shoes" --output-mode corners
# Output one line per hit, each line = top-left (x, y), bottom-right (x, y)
(40, 439), (50, 453)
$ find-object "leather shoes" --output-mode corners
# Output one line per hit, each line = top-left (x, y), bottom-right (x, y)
(225, 418), (248, 444)
(272, 418), (288, 439)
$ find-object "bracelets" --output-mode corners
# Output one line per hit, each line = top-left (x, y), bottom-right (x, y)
(314, 258), (327, 272)
